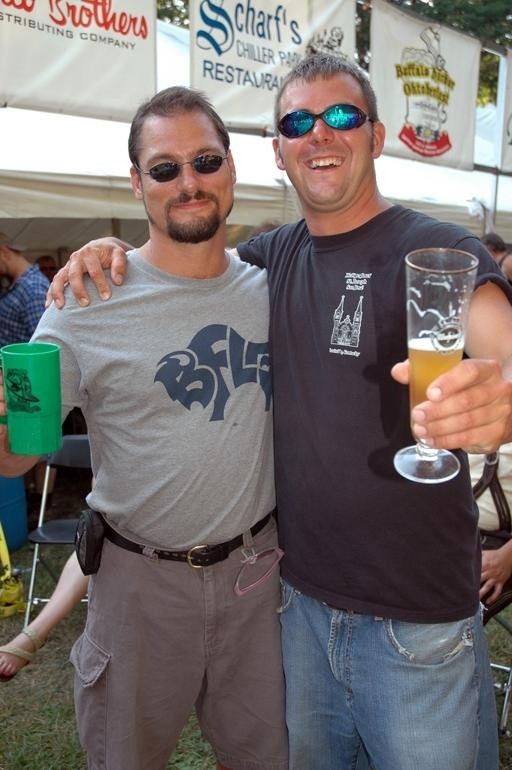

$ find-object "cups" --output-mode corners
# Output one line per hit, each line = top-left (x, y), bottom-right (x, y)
(2, 341), (64, 459)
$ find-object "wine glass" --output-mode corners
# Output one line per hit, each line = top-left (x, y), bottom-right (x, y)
(392, 246), (478, 483)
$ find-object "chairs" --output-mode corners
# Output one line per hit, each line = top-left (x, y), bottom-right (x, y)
(23, 435), (94, 636)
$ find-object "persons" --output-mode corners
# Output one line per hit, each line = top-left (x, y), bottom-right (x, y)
(44, 56), (512, 770)
(466, 442), (512, 630)
(0, 237), (57, 514)
(35, 255), (57, 281)
(481, 232), (512, 284)
(0, 86), (293, 770)
(0, 549), (90, 678)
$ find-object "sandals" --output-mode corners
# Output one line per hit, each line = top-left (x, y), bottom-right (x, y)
(0, 627), (46, 683)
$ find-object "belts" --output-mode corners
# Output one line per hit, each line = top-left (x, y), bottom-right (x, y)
(99, 513), (271, 569)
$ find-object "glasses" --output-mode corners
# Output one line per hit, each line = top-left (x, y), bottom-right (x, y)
(132, 150), (229, 184)
(278, 102), (370, 139)
